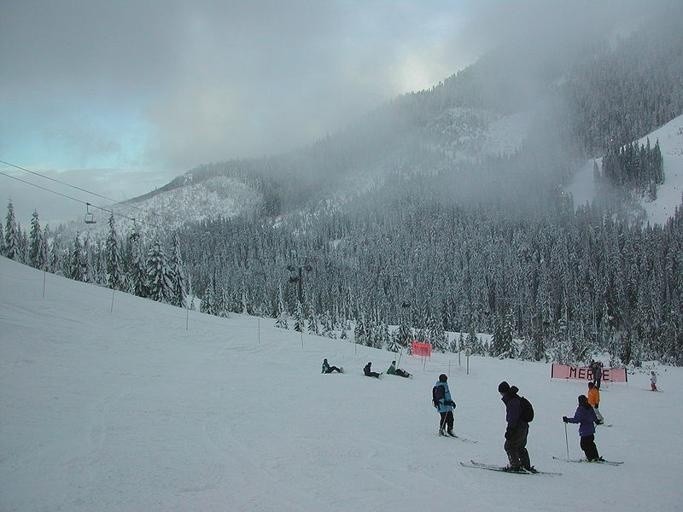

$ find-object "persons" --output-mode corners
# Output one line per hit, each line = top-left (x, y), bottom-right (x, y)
(587, 358), (603, 390)
(385, 360), (409, 377)
(648, 370), (658, 391)
(585, 381), (605, 424)
(497, 380), (530, 472)
(562, 394), (605, 463)
(320, 357), (343, 374)
(433, 372), (458, 438)
(362, 361), (383, 379)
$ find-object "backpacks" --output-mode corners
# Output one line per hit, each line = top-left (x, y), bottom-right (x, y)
(433, 385), (445, 403)
(508, 395), (534, 422)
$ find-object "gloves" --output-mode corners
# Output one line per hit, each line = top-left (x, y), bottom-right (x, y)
(562, 416), (569, 423)
(504, 432), (511, 440)
(595, 419), (603, 425)
(594, 404), (598, 409)
(450, 400), (456, 409)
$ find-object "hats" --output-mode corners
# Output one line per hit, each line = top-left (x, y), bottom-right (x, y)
(439, 373), (447, 382)
(578, 395), (588, 403)
(497, 381), (510, 392)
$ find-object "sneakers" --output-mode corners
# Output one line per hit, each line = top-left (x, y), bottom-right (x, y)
(438, 428), (456, 437)
(586, 458), (600, 462)
(505, 462), (531, 472)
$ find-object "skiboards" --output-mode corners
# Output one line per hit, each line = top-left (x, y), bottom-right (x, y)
(553, 457), (622, 466)
(448, 438), (479, 444)
(460, 461), (564, 475)
